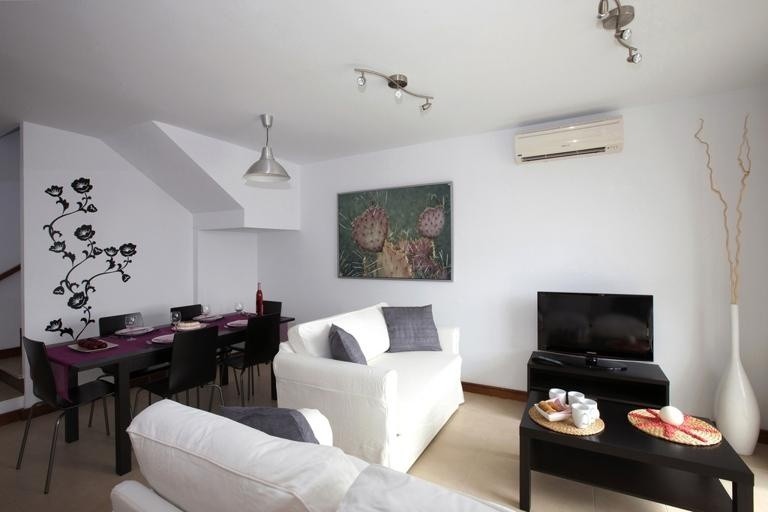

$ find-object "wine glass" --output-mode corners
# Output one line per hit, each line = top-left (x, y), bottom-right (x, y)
(233, 302), (243, 319)
(124, 312), (136, 341)
(170, 311), (182, 333)
(200, 303), (209, 324)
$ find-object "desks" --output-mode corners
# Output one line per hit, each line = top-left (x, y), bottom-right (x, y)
(527, 352), (669, 409)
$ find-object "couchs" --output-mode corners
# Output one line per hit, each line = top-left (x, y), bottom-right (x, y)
(273, 326), (466, 473)
(110, 408), (518, 511)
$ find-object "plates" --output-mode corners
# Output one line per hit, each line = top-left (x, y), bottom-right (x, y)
(68, 339), (118, 352)
(172, 322), (206, 331)
(151, 334), (174, 343)
(114, 326), (154, 336)
(226, 319), (248, 328)
(192, 314), (222, 322)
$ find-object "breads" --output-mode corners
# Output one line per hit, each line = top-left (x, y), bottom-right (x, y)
(538, 401), (564, 414)
(77, 337), (107, 350)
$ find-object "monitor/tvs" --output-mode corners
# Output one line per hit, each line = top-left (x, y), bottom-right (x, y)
(537, 290), (653, 371)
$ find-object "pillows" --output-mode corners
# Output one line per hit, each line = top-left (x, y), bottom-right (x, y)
(216, 406), (320, 444)
(126, 398), (358, 511)
(328, 323), (367, 364)
(338, 462), (516, 511)
(289, 302), (390, 357)
(381, 305), (443, 353)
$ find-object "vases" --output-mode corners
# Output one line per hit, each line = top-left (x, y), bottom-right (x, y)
(716, 301), (759, 454)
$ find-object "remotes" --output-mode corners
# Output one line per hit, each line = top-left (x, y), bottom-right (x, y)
(533, 356), (560, 366)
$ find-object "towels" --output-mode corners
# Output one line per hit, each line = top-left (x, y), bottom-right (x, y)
(177, 322), (201, 329)
(229, 319), (248, 326)
(154, 334), (174, 343)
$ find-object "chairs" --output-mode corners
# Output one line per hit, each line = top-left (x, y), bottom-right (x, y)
(88, 312), (145, 425)
(208, 312), (281, 412)
(255, 300), (282, 376)
(134, 324), (224, 415)
(16, 336), (117, 495)
(170, 304), (202, 322)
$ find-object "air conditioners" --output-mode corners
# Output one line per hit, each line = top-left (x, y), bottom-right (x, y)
(514, 115), (625, 162)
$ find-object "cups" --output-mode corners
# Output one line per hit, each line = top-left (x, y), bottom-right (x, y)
(549, 388), (567, 406)
(579, 398), (600, 423)
(571, 403), (591, 429)
(567, 391), (585, 410)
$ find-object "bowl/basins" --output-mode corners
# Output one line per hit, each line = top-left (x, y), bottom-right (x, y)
(537, 398), (570, 422)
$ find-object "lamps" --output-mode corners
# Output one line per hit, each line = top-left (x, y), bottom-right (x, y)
(598, 0), (643, 63)
(245, 114), (289, 179)
(355, 69), (434, 111)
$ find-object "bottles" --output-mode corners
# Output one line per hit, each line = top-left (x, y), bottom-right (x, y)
(255, 282), (264, 316)
